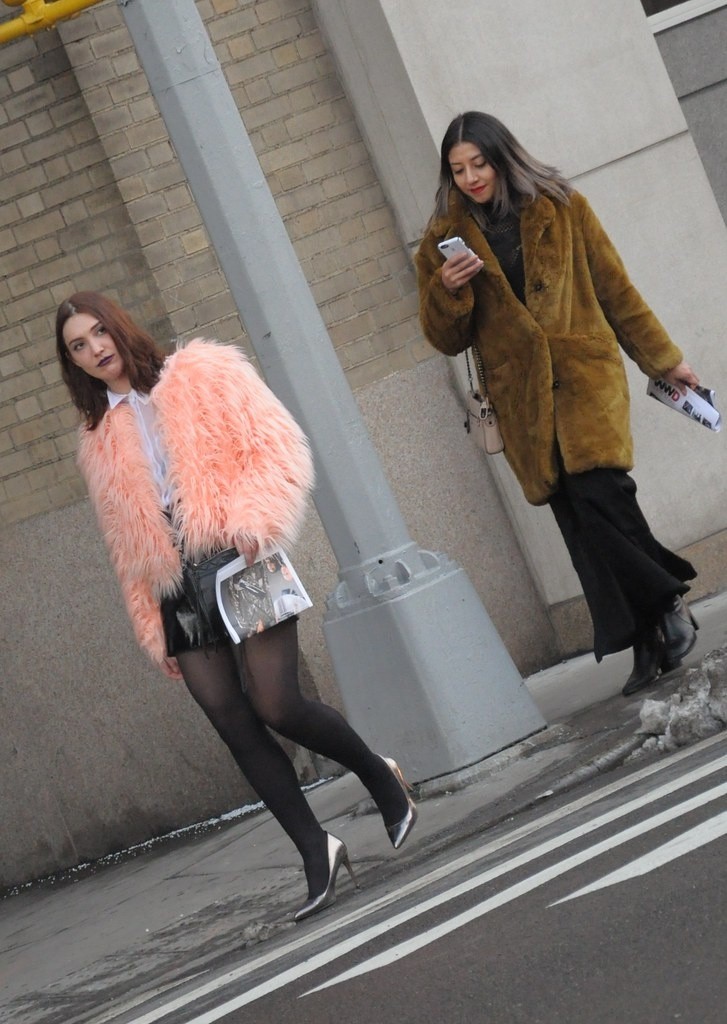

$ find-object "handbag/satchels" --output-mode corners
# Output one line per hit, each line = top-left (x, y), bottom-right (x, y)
(464, 390), (506, 454)
(181, 546), (264, 650)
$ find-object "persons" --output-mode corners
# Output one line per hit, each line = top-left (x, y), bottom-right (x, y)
(264, 554), (292, 582)
(413, 110), (699, 698)
(56, 291), (418, 922)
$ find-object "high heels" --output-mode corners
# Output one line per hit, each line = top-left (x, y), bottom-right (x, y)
(294, 831), (360, 923)
(378, 754), (419, 851)
(622, 609), (672, 699)
(661, 594), (699, 663)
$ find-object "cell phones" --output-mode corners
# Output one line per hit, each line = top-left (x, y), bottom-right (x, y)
(438, 237), (484, 271)
